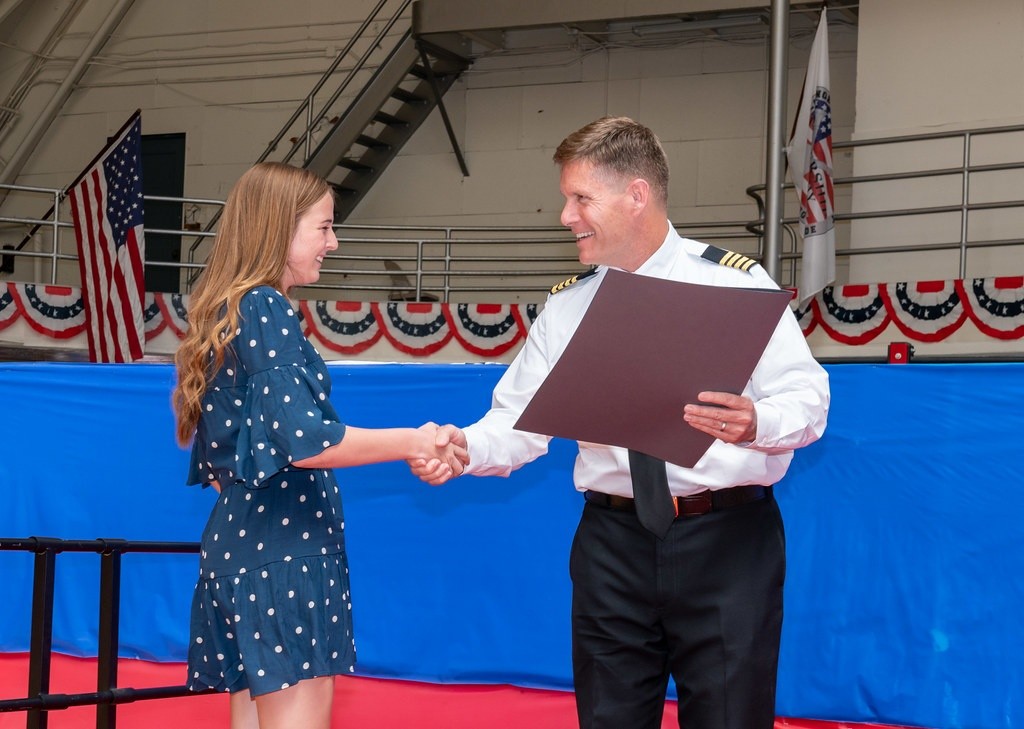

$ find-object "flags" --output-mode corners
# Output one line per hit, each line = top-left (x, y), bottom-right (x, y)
(783, 6), (836, 314)
(66, 115), (145, 363)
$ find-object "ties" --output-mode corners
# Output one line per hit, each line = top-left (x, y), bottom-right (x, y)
(628, 448), (676, 541)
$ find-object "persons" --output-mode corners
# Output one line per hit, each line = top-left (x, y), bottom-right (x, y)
(406, 114), (830, 729)
(172, 162), (470, 729)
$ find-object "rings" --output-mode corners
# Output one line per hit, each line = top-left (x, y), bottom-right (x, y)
(720, 422), (726, 432)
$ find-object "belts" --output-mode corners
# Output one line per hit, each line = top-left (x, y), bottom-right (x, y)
(585, 485), (773, 518)
